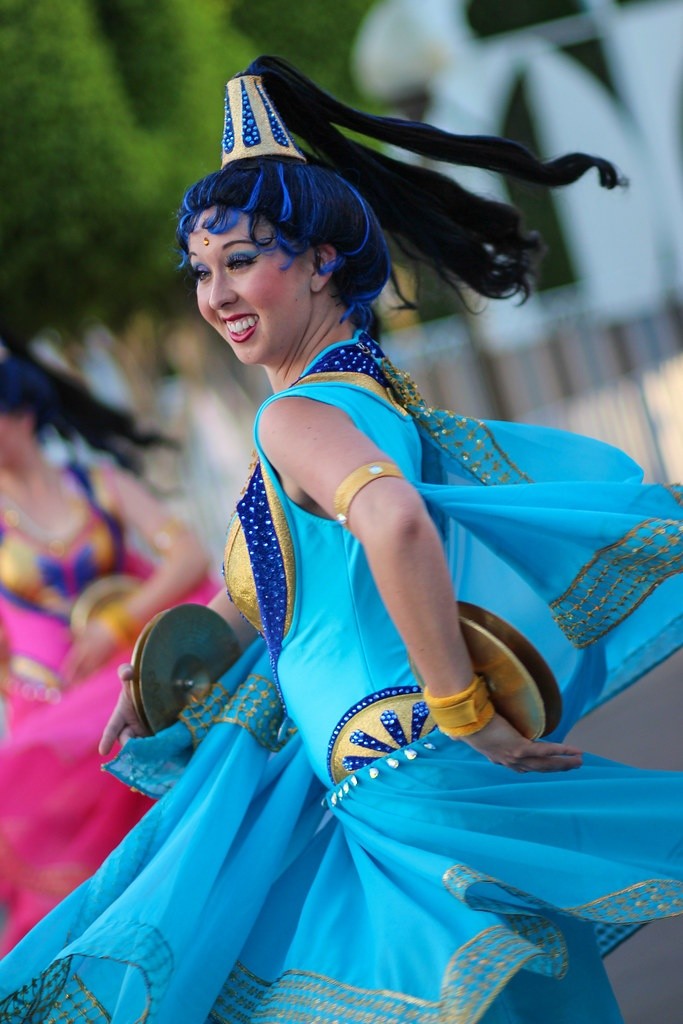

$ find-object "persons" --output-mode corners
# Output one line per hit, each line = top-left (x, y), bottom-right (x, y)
(0, 56), (683, 1024)
(0, 325), (225, 955)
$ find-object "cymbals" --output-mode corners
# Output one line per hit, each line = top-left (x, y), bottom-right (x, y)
(129, 603), (242, 733)
(458, 599), (562, 740)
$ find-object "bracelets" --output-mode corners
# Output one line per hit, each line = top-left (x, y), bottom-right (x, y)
(423, 674), (495, 738)
(98, 602), (141, 648)
